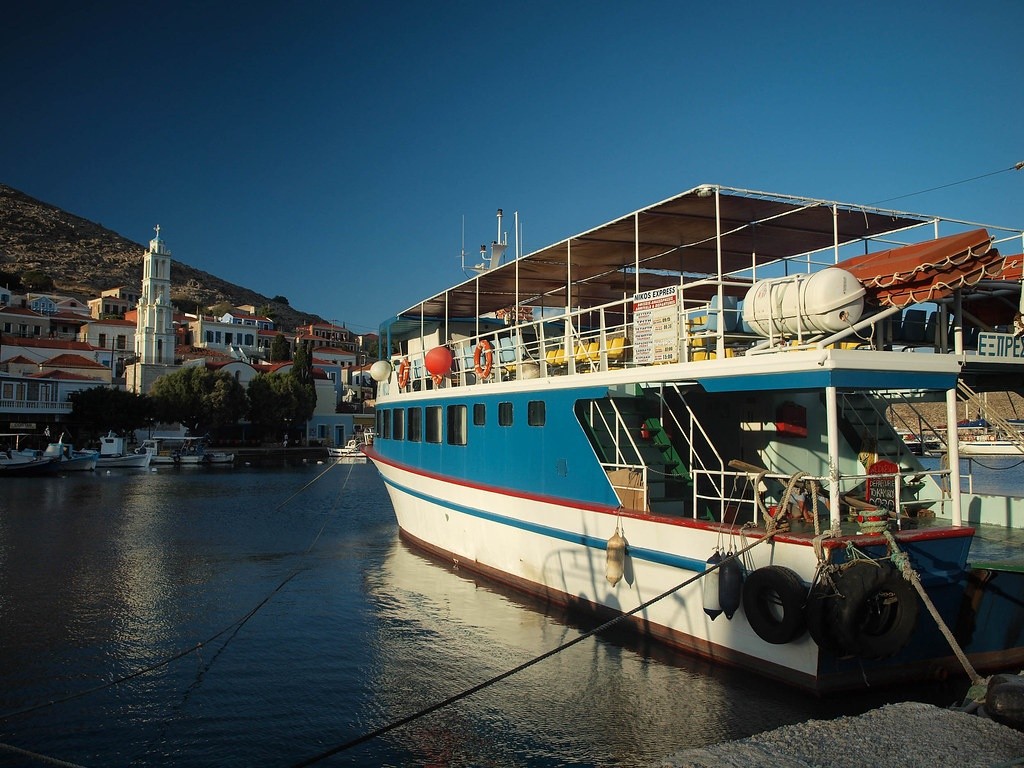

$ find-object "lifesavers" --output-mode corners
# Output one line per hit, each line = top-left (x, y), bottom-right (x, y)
(741, 565), (808, 644)
(398, 359), (409, 389)
(474, 340), (493, 379)
(174, 456), (181, 463)
(808, 561), (917, 661)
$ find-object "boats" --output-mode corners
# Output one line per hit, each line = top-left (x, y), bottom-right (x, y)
(902, 417), (1024, 456)
(135, 435), (234, 463)
(11, 432), (99, 471)
(97, 431), (152, 467)
(327, 440), (367, 457)
(357, 183), (1023, 706)
(0, 451), (52, 469)
